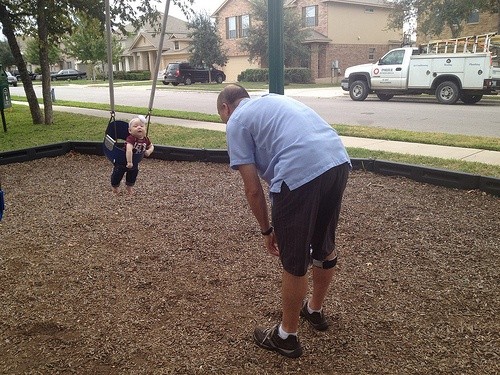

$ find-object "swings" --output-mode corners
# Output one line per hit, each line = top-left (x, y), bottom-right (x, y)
(104, 0), (169, 162)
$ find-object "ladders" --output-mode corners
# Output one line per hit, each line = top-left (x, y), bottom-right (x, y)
(417, 32), (498, 58)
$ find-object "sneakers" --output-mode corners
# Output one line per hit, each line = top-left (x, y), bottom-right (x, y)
(254, 322), (303, 358)
(300, 302), (329, 330)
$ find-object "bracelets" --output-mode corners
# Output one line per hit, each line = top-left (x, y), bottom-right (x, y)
(260, 226), (274, 235)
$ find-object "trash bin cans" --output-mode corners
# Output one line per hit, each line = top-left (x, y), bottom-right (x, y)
(0, 73), (12, 109)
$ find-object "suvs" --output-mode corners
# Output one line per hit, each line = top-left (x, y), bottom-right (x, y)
(10, 69), (36, 81)
(5, 72), (17, 87)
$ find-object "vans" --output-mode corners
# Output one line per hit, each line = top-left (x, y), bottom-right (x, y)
(163, 62), (227, 86)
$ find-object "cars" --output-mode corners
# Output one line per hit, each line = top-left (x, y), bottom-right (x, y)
(50, 68), (87, 81)
(35, 71), (58, 80)
(157, 69), (166, 84)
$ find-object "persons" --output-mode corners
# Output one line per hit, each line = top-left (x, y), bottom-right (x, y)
(217, 84), (352, 357)
(110, 115), (155, 194)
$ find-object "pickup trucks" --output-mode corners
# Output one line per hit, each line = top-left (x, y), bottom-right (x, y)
(340, 46), (500, 104)
